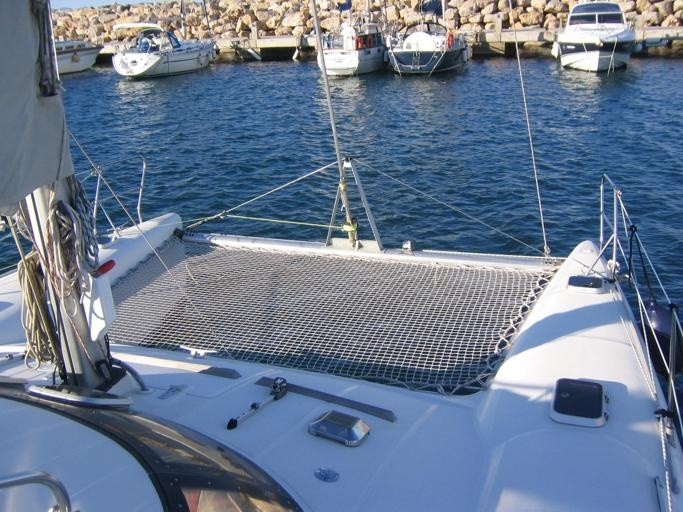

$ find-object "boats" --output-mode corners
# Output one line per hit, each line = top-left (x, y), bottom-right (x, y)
(315, 0), (389, 76)
(54, 39), (104, 75)
(0, 0), (683, 508)
(384, 0), (473, 77)
(549, 0), (636, 72)
(110, 1), (217, 80)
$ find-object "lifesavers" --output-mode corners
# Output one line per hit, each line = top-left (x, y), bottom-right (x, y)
(367, 35), (373, 49)
(358, 37), (363, 49)
(449, 33), (453, 48)
